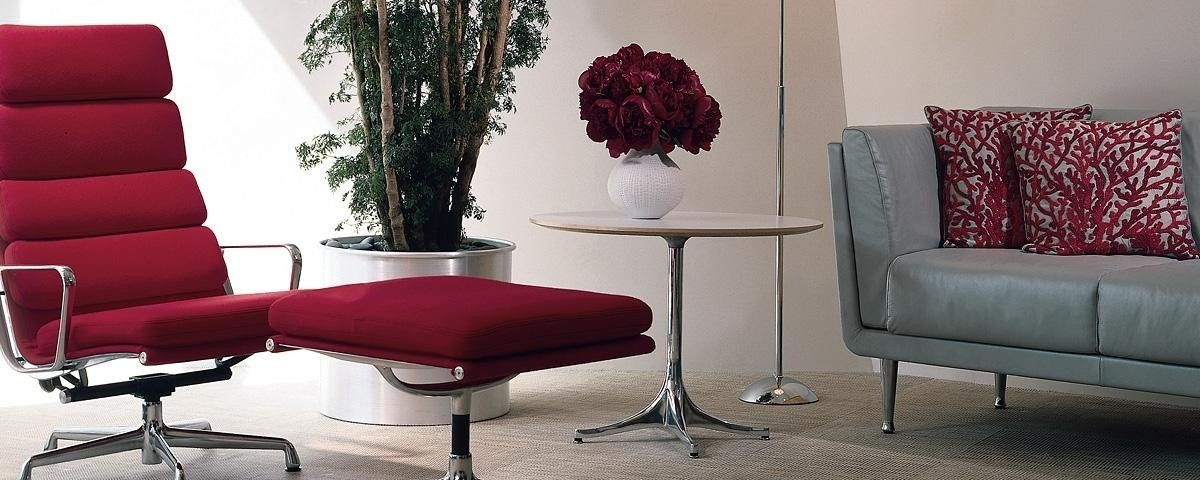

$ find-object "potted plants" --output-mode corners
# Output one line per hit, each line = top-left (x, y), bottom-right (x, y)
(294, 0), (552, 428)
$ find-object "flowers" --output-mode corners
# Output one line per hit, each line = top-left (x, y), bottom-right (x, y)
(577, 41), (723, 158)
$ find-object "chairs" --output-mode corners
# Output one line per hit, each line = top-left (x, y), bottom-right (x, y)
(2, 23), (303, 480)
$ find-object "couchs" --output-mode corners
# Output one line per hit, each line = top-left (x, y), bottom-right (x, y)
(820, 107), (1200, 433)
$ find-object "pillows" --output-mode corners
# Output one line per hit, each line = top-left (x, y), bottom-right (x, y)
(923, 106), (1094, 249)
(1005, 109), (1200, 259)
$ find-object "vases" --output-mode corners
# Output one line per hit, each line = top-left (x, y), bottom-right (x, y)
(606, 154), (689, 221)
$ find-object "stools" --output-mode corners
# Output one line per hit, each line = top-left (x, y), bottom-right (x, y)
(266, 276), (656, 480)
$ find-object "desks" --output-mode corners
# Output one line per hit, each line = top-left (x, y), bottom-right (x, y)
(529, 209), (824, 459)
